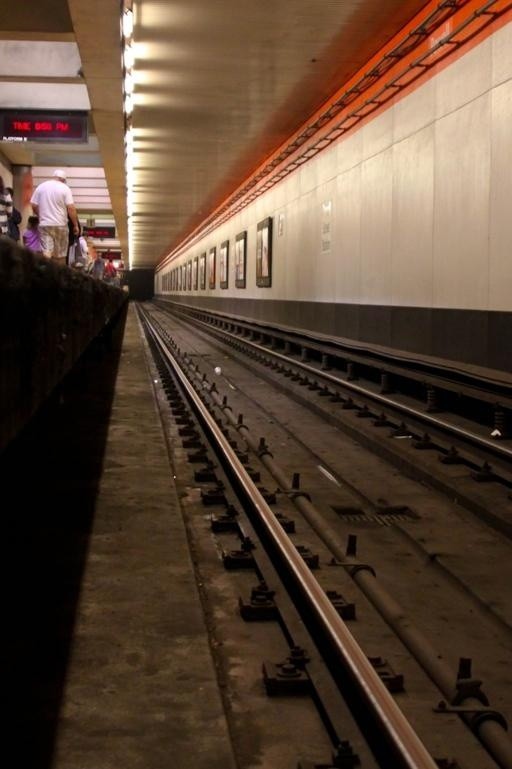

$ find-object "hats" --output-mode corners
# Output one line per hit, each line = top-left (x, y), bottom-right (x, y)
(52, 169), (67, 181)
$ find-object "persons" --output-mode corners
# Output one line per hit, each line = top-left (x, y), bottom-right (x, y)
(1, 169), (124, 286)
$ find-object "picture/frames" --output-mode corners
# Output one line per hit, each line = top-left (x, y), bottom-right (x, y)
(162, 216), (273, 291)
